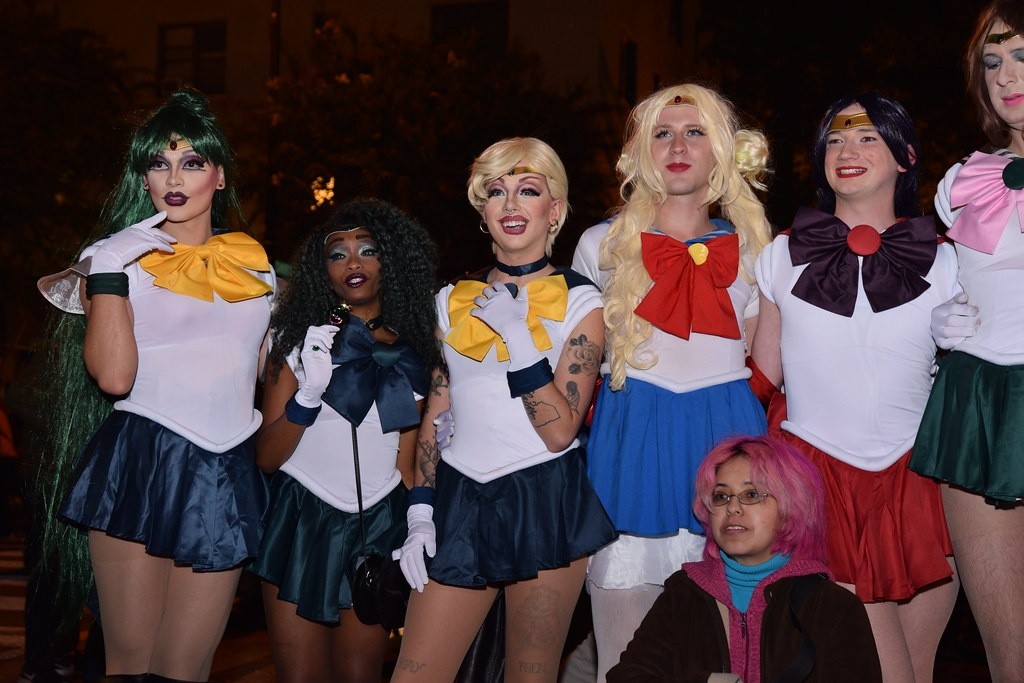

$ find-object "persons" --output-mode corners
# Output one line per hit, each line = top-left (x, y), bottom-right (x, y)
(253, 198), (425, 683)
(926, 1), (1024, 683)
(586, 93), (963, 683)
(36, 90), (278, 683)
(603, 435), (883, 683)
(433, 84), (775, 683)
(389, 138), (604, 683)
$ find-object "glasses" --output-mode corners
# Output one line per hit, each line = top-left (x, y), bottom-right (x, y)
(711, 488), (774, 506)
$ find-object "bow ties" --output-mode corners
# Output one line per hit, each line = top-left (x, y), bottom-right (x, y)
(635, 231), (742, 340)
(137, 229), (272, 303)
(322, 313), (433, 433)
(946, 150), (1024, 255)
(445, 274), (571, 363)
(787, 209), (939, 318)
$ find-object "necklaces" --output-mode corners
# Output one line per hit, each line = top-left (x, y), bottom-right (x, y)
(362, 313), (382, 331)
(496, 252), (548, 277)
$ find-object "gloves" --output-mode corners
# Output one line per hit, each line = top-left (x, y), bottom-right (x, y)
(87, 210), (178, 274)
(930, 294), (981, 350)
(470, 280), (545, 372)
(399, 504), (436, 593)
(295, 324), (339, 407)
